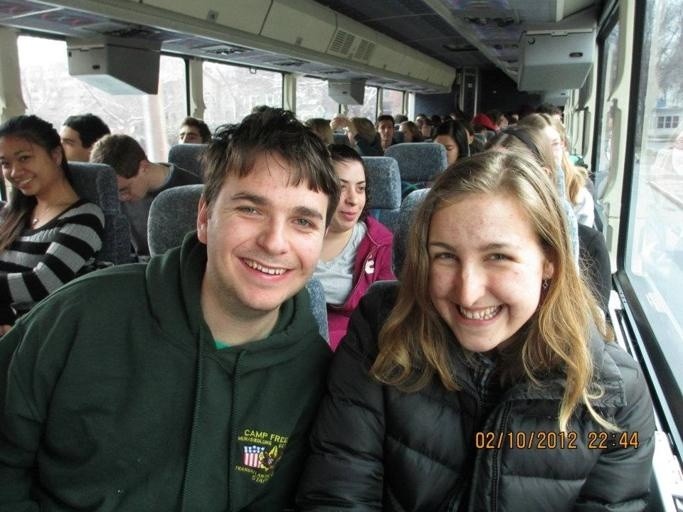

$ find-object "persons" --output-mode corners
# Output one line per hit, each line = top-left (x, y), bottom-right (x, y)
(288, 147), (657, 511)
(1, 105), (342, 512)
(0, 114), (106, 340)
(61, 101), (610, 352)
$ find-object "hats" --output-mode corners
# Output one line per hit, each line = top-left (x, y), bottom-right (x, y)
(471, 113), (496, 132)
